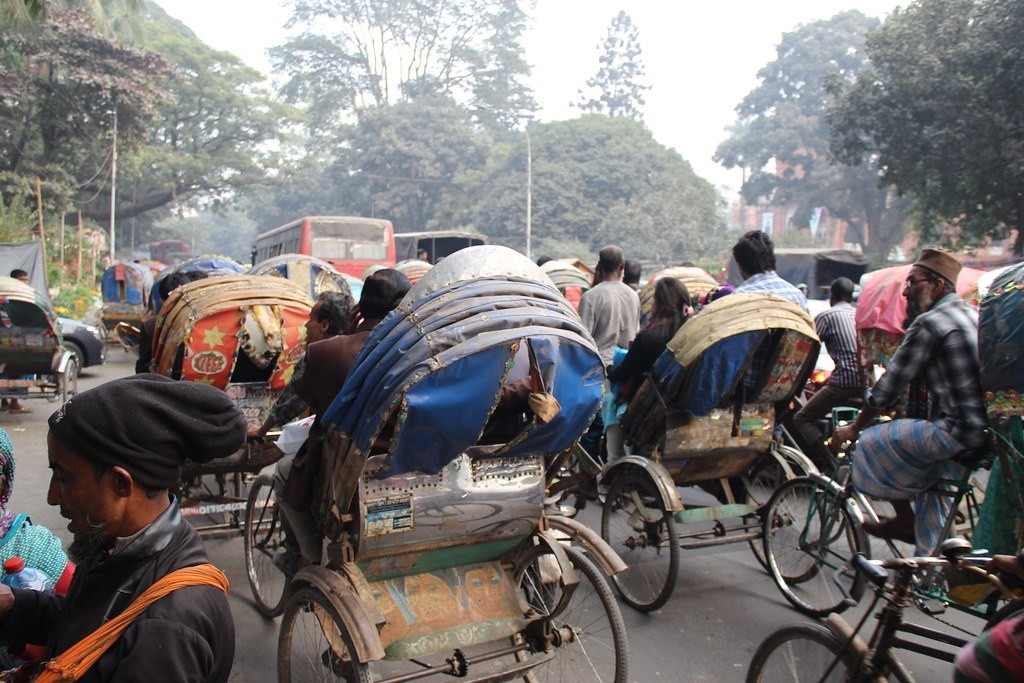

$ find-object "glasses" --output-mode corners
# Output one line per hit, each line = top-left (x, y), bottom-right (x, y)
(907, 278), (941, 290)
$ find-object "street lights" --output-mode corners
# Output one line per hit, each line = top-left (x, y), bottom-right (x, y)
(476, 104), (532, 261)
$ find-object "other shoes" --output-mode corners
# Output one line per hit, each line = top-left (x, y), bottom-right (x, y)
(861, 519), (919, 545)
(816, 448), (837, 471)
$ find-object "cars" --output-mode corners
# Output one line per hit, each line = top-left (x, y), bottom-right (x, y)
(37, 315), (106, 384)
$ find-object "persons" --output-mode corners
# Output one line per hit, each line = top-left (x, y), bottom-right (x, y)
(951, 546), (1024, 683)
(576, 244), (695, 505)
(833, 248), (999, 580)
(0, 426), (77, 662)
(793, 277), (873, 477)
(417, 248), (429, 263)
(732, 231), (811, 313)
(135, 271), (211, 374)
(246, 269), (532, 569)
(0, 370), (255, 683)
(0, 269), (33, 414)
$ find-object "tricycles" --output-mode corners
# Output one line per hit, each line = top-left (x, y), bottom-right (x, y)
(143, 245), (681, 683)
(0, 276), (78, 406)
(547, 267), (830, 612)
(93, 258), (167, 351)
(745, 250), (1024, 682)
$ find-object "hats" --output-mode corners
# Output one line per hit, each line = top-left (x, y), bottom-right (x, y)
(47, 370), (248, 489)
(913, 247), (962, 287)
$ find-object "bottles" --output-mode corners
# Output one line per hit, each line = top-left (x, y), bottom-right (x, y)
(2, 556), (55, 598)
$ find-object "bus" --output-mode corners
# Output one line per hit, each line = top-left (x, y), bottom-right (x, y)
(151, 240), (193, 266)
(252, 216), (396, 282)
(392, 232), (489, 267)
